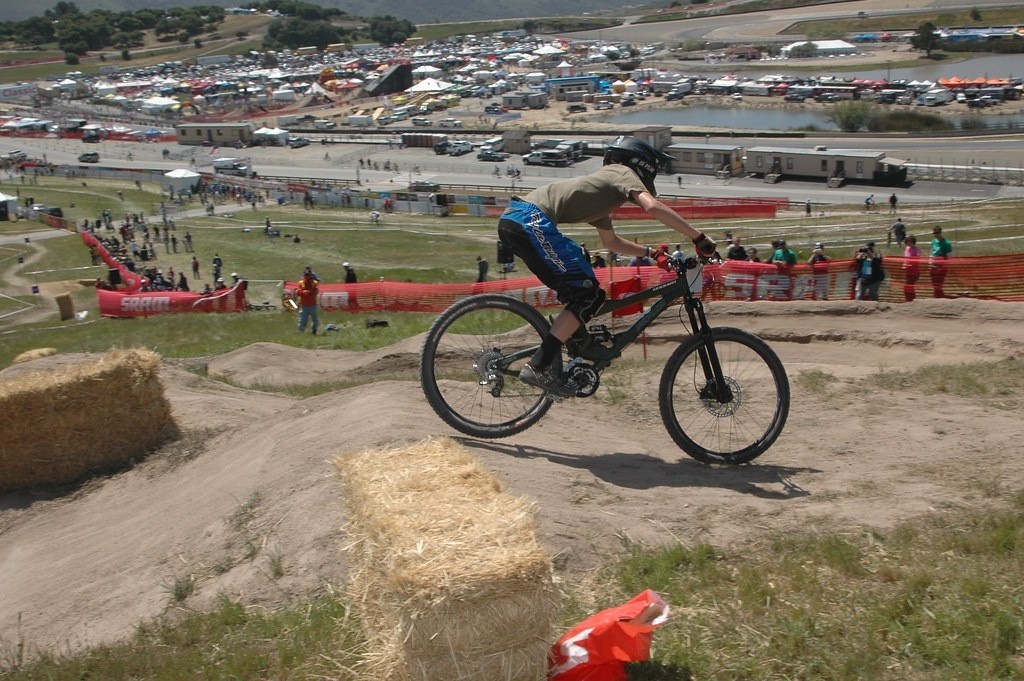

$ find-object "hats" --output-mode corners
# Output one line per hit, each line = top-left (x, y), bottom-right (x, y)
(771, 239), (778, 243)
(217, 278), (223, 282)
(815, 243), (823, 248)
(343, 262), (349, 266)
(779, 240), (784, 244)
(213, 264), (216, 266)
(660, 243), (668, 250)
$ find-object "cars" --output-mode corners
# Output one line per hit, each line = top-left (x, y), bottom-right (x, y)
(78, 152), (100, 163)
(0, 85), (1023, 219)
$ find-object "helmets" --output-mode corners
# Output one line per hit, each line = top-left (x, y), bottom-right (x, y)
(603, 136), (676, 196)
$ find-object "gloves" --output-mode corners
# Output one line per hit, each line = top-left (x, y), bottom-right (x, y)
(692, 232), (716, 260)
(653, 251), (671, 272)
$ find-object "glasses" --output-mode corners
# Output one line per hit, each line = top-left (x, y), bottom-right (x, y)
(933, 233), (939, 234)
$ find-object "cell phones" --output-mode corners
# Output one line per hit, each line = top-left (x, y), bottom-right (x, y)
(860, 248), (867, 252)
(815, 243), (821, 249)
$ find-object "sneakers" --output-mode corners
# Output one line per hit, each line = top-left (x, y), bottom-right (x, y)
(566, 338), (622, 362)
(519, 361), (575, 397)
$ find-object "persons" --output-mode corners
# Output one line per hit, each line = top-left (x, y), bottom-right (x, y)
(471, 226), (953, 301)
(293, 266), (321, 334)
(498, 136), (715, 397)
(865, 194), (874, 211)
(805, 198), (812, 217)
(886, 217), (906, 248)
(168, 180), (271, 216)
(80, 201), (249, 298)
(888, 192), (898, 214)
(342, 261), (359, 310)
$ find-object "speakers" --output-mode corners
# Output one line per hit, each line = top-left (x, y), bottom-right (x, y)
(498, 241), (514, 263)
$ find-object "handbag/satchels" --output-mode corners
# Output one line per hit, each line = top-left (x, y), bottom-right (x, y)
(548, 589), (669, 681)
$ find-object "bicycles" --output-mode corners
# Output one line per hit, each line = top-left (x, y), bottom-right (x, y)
(418, 236), (792, 466)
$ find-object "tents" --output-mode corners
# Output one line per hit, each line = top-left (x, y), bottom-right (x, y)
(0, 33), (1024, 179)
